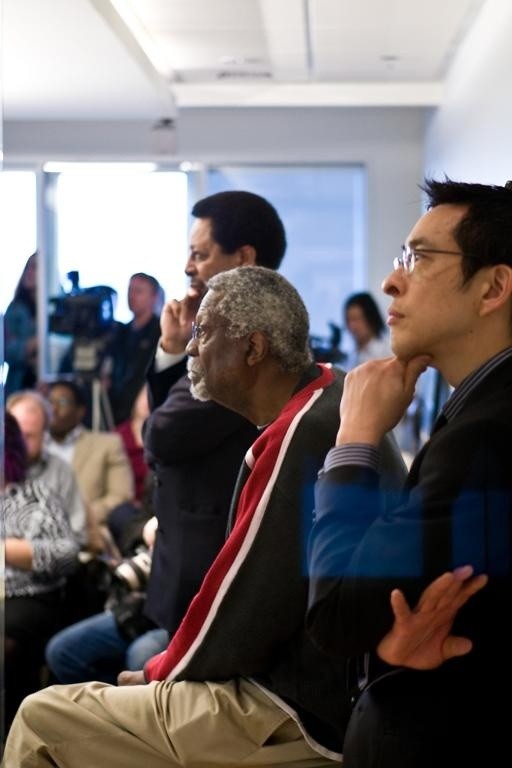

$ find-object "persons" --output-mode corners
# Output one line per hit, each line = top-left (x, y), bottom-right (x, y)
(2, 172), (511, 766)
(342, 293), (408, 451)
(1, 189), (403, 757)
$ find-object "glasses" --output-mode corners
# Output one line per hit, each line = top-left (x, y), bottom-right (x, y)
(390, 246), (484, 274)
(190, 321), (240, 340)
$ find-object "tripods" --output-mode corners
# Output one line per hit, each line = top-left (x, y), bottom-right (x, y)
(68, 340), (116, 432)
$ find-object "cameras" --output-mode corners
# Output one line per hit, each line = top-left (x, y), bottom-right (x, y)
(112, 543), (153, 591)
